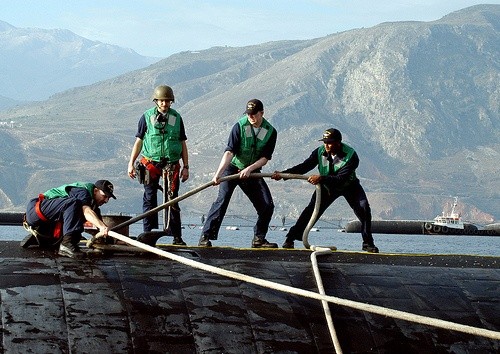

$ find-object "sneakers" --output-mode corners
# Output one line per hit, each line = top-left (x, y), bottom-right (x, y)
(19, 233), (36, 248)
(58, 242), (87, 260)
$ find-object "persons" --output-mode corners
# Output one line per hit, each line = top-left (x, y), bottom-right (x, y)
(20, 180), (117, 259)
(197, 99), (278, 248)
(128, 85), (189, 246)
(272, 128), (379, 253)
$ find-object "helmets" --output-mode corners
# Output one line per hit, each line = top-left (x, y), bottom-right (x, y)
(151, 85), (174, 102)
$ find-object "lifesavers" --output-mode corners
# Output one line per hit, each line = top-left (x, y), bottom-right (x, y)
(487, 230), (492, 235)
(455, 229), (459, 233)
(479, 230), (483, 234)
(492, 231), (496, 235)
(460, 230), (463, 234)
(450, 228), (454, 233)
(442, 227), (449, 232)
(464, 229), (468, 234)
(425, 223), (432, 230)
(469, 229), (473, 234)
(434, 226), (440, 232)
(474, 229), (478, 234)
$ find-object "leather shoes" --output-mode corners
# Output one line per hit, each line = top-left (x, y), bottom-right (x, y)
(198, 234), (213, 247)
(252, 235), (279, 248)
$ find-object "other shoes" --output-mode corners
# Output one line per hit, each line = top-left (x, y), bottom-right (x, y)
(362, 239), (379, 253)
(283, 237), (295, 248)
(174, 236), (187, 245)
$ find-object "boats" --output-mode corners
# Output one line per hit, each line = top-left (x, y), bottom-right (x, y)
(431, 195), (464, 230)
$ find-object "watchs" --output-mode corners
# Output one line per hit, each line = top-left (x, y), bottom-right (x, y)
(183, 166), (189, 169)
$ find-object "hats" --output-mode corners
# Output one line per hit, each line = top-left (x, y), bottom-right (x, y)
(317, 128), (342, 142)
(94, 180), (117, 200)
(242, 99), (264, 115)
